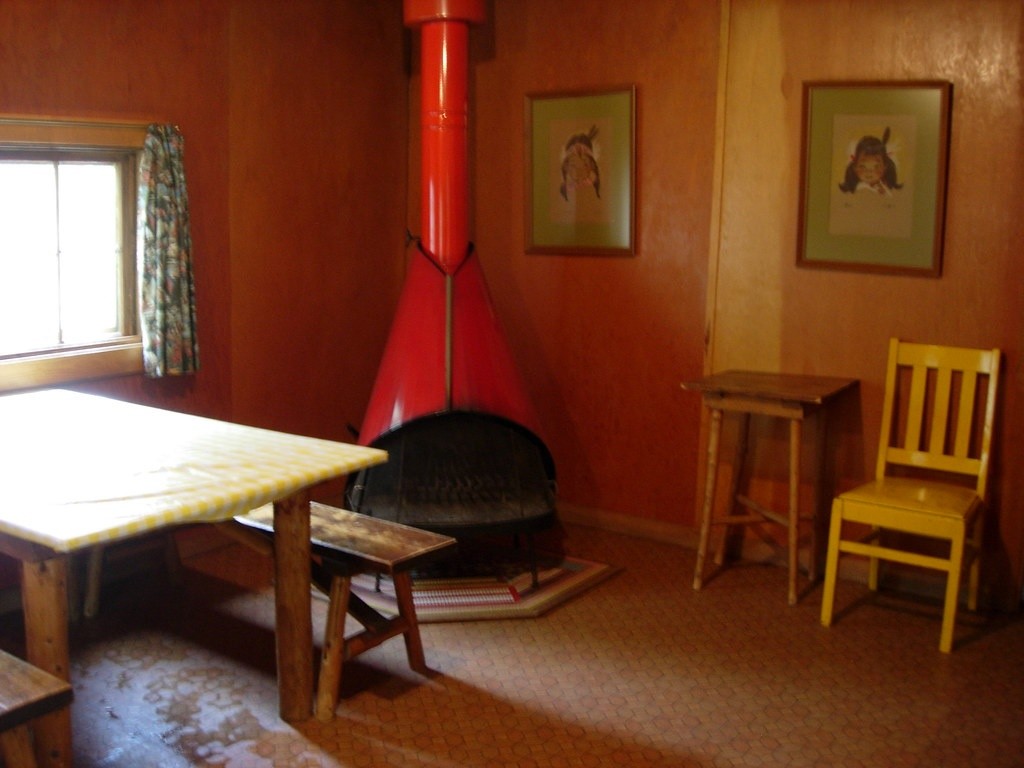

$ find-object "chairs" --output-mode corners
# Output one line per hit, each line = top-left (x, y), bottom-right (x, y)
(820, 337), (1002, 653)
(347, 409), (558, 593)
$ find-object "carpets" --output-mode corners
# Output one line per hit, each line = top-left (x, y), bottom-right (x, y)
(346, 536), (623, 623)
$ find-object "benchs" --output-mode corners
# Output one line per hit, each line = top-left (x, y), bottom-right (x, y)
(0, 648), (75, 768)
(85, 500), (457, 726)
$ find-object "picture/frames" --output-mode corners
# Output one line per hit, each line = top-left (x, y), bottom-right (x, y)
(795, 79), (953, 279)
(520, 82), (640, 258)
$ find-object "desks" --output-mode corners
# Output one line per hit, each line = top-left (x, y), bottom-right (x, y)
(680, 368), (860, 605)
(0, 387), (389, 768)
